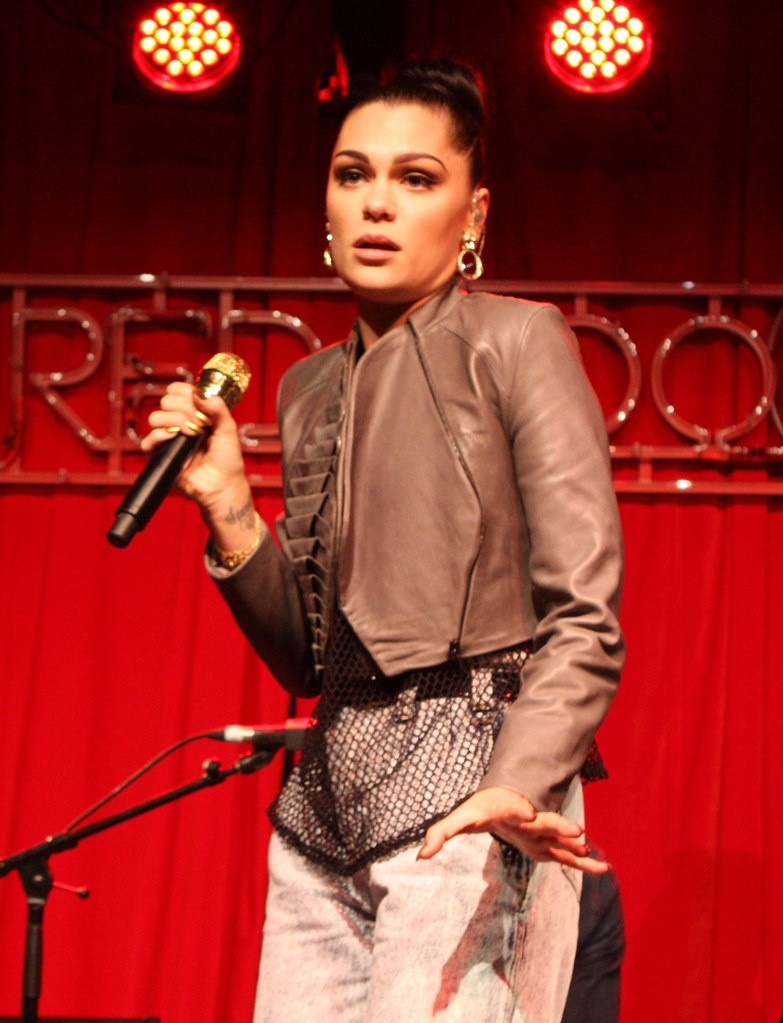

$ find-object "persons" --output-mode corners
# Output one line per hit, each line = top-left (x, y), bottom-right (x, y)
(141, 56), (626, 1022)
(562, 842), (623, 1022)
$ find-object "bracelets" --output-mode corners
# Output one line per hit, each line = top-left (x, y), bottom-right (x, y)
(214, 510), (261, 568)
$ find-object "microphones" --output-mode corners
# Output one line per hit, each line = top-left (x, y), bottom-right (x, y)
(104, 351), (251, 548)
(208, 718), (316, 750)
